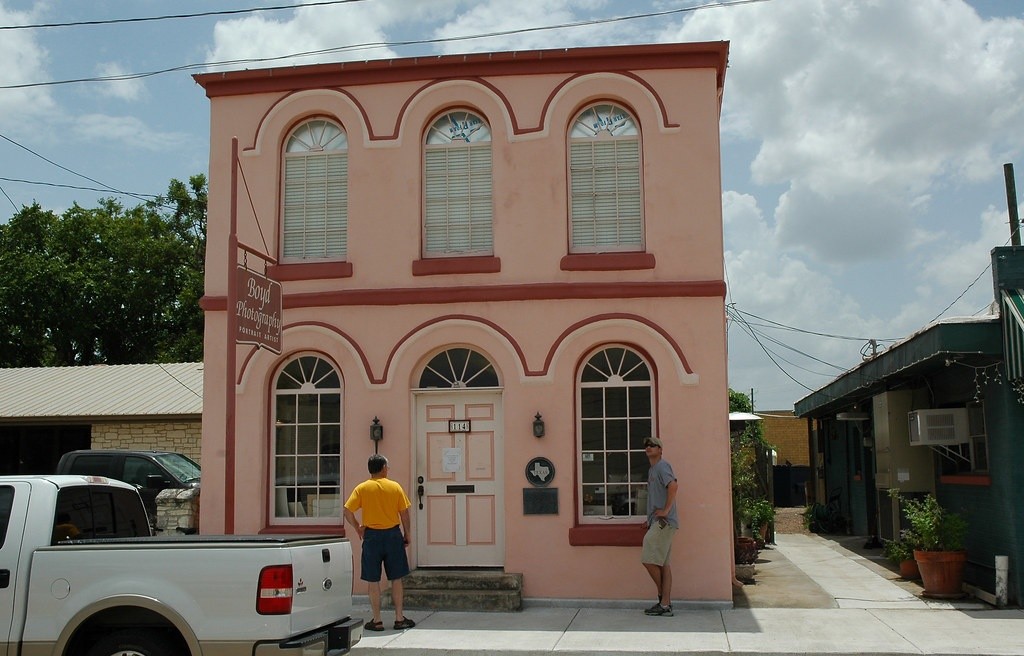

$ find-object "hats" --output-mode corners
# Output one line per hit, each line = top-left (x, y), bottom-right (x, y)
(643, 437), (663, 448)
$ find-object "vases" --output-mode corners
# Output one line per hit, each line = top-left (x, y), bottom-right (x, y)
(734, 564), (756, 585)
(734, 537), (759, 564)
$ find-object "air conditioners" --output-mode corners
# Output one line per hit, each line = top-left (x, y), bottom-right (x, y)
(907, 408), (969, 447)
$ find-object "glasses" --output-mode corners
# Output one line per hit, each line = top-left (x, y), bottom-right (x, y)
(645, 443), (660, 448)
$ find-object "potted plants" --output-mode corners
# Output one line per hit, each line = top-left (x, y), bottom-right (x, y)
(738, 499), (779, 548)
(880, 487), (972, 600)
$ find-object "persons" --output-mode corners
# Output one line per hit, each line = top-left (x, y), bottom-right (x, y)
(641, 436), (681, 617)
(342, 454), (416, 631)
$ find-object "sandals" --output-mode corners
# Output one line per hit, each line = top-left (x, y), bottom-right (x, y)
(364, 619), (385, 631)
(394, 615), (415, 630)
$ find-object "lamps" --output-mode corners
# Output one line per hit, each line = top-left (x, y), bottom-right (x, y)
(532, 412), (545, 438)
(368, 416), (383, 455)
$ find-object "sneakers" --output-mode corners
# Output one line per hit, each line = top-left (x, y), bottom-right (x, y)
(644, 593), (674, 617)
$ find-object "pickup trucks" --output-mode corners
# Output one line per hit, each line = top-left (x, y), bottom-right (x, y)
(56, 448), (201, 530)
(0, 474), (365, 656)
